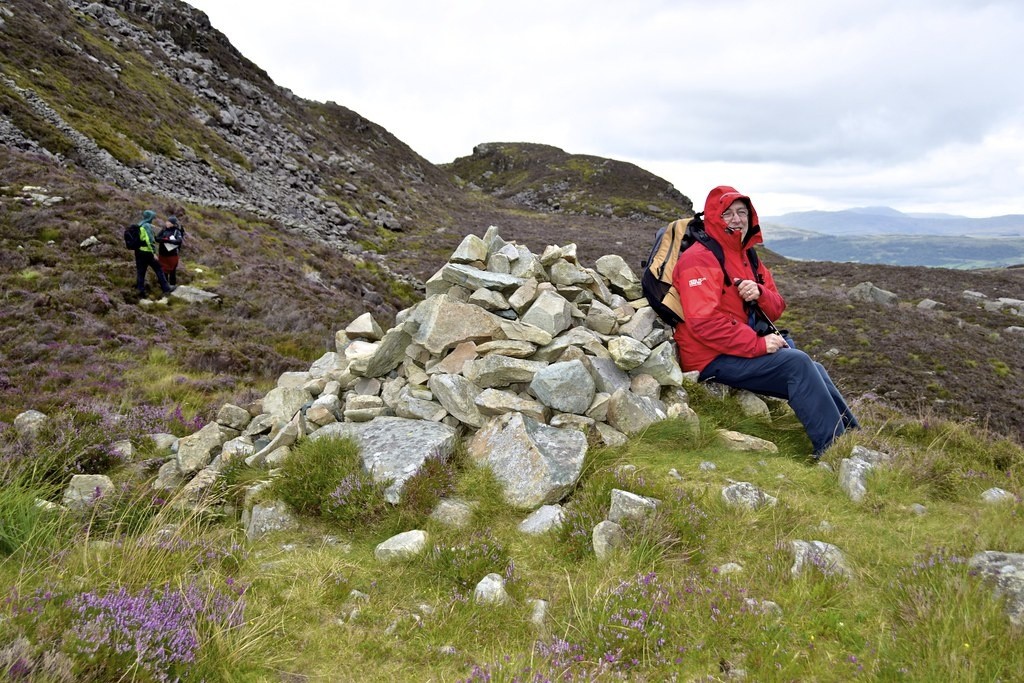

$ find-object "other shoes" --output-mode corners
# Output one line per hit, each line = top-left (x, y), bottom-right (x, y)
(163, 285), (177, 294)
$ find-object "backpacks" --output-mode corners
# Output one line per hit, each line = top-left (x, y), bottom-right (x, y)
(124, 222), (141, 250)
(640, 212), (704, 328)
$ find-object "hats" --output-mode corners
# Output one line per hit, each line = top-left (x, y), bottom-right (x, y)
(167, 216), (178, 225)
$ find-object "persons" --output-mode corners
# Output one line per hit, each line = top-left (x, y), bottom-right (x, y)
(155, 215), (183, 286)
(134, 210), (176, 299)
(671, 185), (890, 461)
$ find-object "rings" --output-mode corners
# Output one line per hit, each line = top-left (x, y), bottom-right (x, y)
(748, 290), (751, 295)
